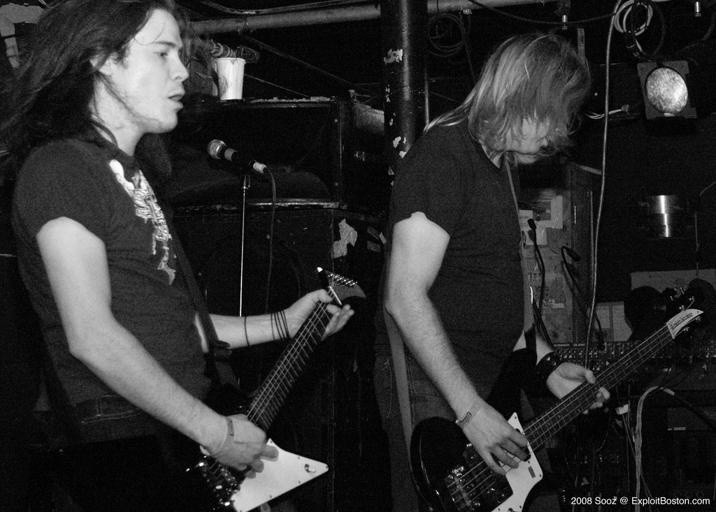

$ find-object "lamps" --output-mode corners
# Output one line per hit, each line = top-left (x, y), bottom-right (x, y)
(633, 55), (714, 122)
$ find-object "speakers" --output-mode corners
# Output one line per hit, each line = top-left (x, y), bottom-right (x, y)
(170, 94), (385, 208)
(170, 208), (392, 512)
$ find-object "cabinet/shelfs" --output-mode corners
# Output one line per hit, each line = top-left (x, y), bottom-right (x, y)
(166, 89), (394, 512)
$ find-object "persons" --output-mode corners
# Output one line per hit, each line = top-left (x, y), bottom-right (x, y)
(381, 30), (610, 510)
(0, 1), (356, 512)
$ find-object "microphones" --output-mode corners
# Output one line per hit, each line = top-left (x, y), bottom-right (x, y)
(561, 246), (603, 345)
(527, 219), (554, 346)
(206, 139), (266, 175)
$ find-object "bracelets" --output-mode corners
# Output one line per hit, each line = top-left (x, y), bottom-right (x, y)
(455, 396), (481, 428)
(199, 413), (236, 458)
(533, 350), (565, 386)
(270, 308), (292, 342)
(243, 314), (251, 347)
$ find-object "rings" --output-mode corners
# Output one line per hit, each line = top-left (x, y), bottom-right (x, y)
(241, 465), (251, 475)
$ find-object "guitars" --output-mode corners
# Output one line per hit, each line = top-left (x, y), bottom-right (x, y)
(411, 295), (705, 512)
(140, 264), (367, 510)
